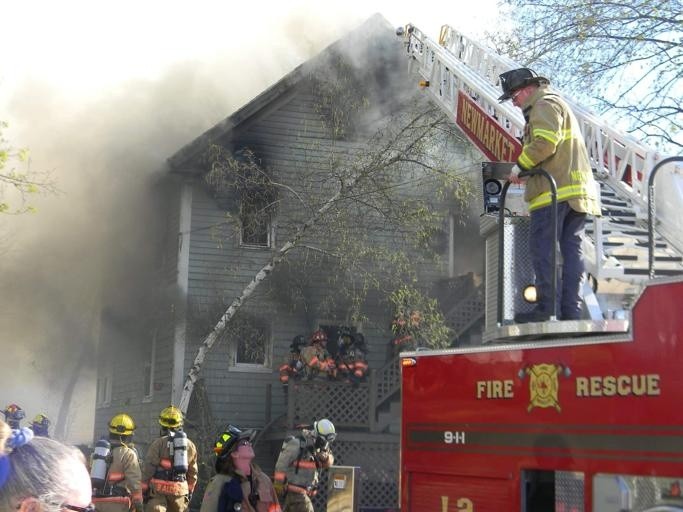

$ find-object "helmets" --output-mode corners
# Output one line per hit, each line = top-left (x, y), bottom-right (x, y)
(293, 330), (364, 346)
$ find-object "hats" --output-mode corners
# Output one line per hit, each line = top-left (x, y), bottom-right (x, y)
(497, 68), (548, 100)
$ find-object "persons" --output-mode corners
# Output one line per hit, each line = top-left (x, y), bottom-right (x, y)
(389, 299), (419, 359)
(286, 326), (367, 384)
(497, 67), (599, 323)
(272, 419), (335, 512)
(89, 414), (141, 512)
(0, 404), (93, 512)
(144, 407), (199, 512)
(201, 424), (281, 511)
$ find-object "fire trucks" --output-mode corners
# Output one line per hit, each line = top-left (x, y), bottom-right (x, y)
(394, 23), (682, 511)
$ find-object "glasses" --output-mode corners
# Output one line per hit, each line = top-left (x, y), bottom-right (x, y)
(512, 90), (523, 100)
